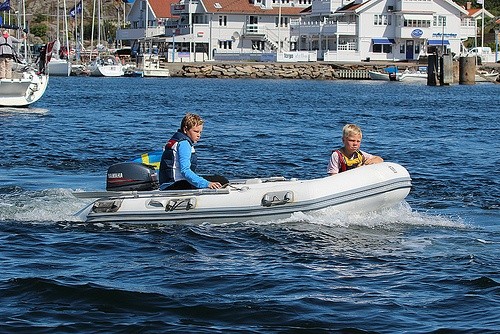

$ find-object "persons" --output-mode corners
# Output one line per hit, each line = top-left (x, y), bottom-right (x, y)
(159, 113), (229, 190)
(38, 46), (45, 75)
(0, 31), (27, 79)
(327, 124), (383, 177)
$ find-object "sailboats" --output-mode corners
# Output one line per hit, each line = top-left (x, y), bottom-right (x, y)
(0, 0), (49, 107)
(125, 0), (172, 78)
(44, 0), (71, 77)
(74, 0), (125, 78)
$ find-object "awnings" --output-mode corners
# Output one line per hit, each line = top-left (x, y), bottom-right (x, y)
(428, 40), (449, 45)
(372, 39), (396, 45)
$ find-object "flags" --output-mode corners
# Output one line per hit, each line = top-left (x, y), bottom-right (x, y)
(70, 2), (83, 18)
(0, 0), (10, 10)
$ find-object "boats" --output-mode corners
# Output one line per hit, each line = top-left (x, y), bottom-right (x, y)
(81, 162), (412, 223)
(368, 15), (499, 84)
